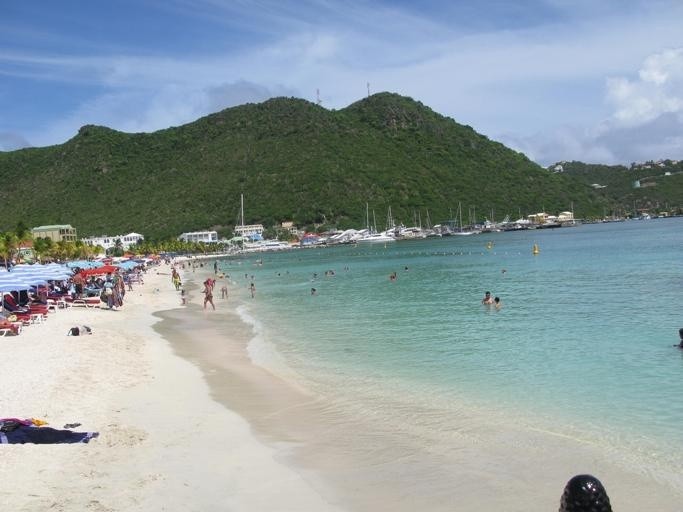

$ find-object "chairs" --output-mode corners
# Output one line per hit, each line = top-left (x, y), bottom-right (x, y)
(0, 266), (102, 341)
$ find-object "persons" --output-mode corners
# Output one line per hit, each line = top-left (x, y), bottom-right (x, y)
(391, 265), (410, 283)
(481, 291), (494, 306)
(673, 328), (683, 349)
(1, 251), (351, 311)
(494, 297), (503, 310)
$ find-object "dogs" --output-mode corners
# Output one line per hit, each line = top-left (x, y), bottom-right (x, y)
(67, 325), (92, 336)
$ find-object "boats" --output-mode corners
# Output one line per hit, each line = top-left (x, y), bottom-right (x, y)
(601, 215), (625, 223)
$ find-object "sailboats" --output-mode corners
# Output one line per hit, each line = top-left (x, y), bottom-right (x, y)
(223, 193), (575, 254)
